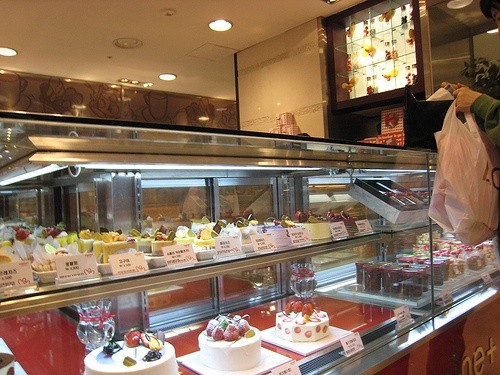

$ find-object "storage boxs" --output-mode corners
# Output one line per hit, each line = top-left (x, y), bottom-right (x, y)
(347, 176), (423, 225)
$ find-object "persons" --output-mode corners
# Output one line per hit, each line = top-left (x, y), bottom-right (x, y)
(182, 186), (240, 216)
(441, 0), (500, 148)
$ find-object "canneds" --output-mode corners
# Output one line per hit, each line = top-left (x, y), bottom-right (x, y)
(354, 253), (449, 298)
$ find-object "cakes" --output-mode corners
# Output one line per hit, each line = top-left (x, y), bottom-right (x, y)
(198, 313), (261, 370)
(84, 330), (180, 375)
(291, 210), (360, 240)
(275, 300), (329, 342)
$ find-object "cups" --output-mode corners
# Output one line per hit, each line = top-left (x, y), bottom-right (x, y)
(74, 301), (116, 350)
(289, 264), (317, 299)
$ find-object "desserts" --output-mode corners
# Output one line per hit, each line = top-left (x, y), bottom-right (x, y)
(0, 215), (297, 285)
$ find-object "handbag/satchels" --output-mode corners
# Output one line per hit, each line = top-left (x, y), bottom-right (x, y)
(427, 97), (500, 245)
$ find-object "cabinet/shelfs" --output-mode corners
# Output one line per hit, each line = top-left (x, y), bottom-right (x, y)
(0, 106), (498, 375)
(344, 0), (421, 97)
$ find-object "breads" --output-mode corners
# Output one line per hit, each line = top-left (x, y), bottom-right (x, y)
(446, 254), (486, 278)
